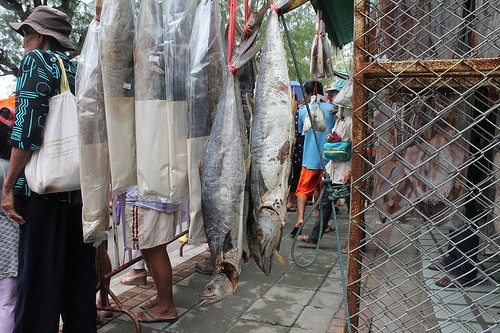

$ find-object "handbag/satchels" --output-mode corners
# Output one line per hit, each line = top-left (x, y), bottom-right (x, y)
(24, 54), (82, 195)
(323, 140), (351, 163)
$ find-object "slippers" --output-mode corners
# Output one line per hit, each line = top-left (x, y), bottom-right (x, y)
(135, 310), (177, 323)
(324, 226), (335, 233)
(140, 300), (155, 310)
(290, 226), (300, 237)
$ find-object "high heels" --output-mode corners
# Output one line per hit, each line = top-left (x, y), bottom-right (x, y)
(120, 270), (147, 287)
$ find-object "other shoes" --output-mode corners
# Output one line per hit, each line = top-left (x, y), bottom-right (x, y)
(434, 274), (479, 288)
(428, 261), (450, 271)
(297, 234), (316, 247)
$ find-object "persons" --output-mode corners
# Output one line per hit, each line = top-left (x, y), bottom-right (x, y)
(429, 133), (485, 287)
(95, 184), (180, 323)
(1, 5), (97, 333)
(287, 79), (354, 254)
(0, 107), (20, 333)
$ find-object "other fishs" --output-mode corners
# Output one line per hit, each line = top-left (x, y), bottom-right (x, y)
(243, 0), (296, 278)
(301, 92), (329, 136)
(363, 0), (500, 224)
(74, 0), (228, 252)
(197, 25), (264, 309)
(307, 21), (336, 79)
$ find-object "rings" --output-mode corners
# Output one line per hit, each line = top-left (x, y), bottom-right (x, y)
(8, 213), (10, 215)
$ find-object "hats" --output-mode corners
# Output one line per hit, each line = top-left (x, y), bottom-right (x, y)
(325, 80), (344, 91)
(0, 99), (15, 127)
(9, 5), (76, 52)
